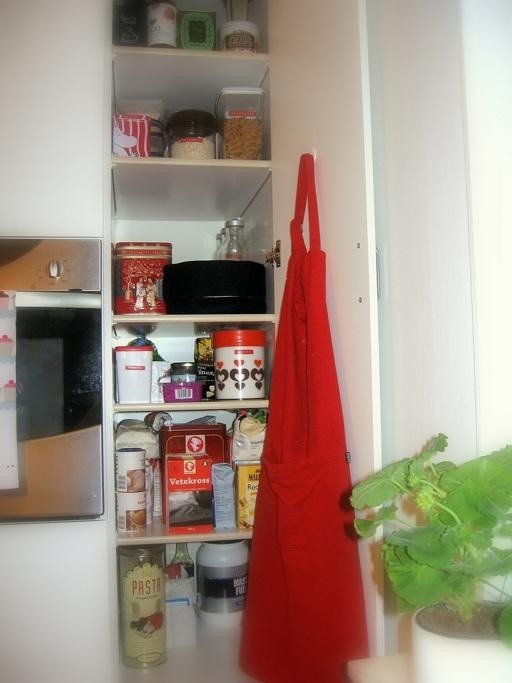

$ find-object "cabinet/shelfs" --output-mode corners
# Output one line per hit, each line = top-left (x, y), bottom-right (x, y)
(110, 0), (386, 683)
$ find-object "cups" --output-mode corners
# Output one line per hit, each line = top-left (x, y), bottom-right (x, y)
(113, 346), (154, 405)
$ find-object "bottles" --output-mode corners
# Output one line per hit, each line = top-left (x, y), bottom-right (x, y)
(213, 216), (250, 260)
(165, 110), (218, 159)
(170, 542), (196, 593)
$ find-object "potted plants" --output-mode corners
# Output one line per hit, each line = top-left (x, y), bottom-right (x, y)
(350, 434), (512, 683)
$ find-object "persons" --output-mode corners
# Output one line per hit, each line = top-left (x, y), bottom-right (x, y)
(144, 276), (160, 310)
(132, 276), (146, 312)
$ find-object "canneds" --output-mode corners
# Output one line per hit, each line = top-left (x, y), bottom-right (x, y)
(115, 448), (147, 493)
(170, 362), (198, 383)
(115, 492), (147, 534)
